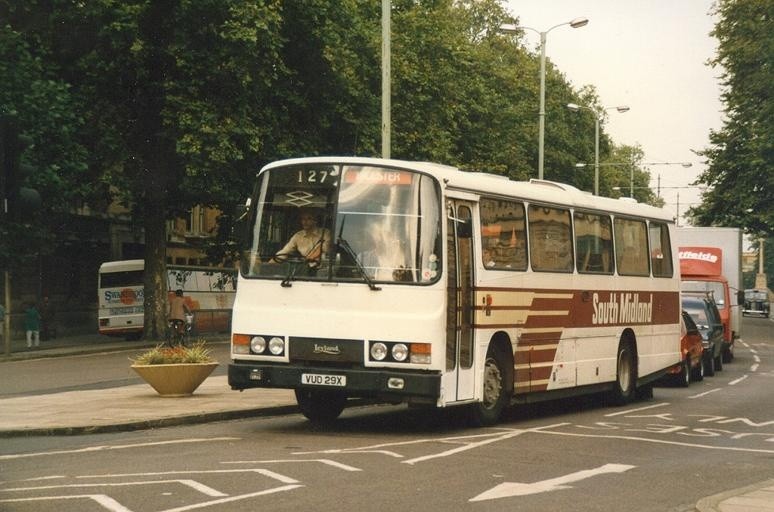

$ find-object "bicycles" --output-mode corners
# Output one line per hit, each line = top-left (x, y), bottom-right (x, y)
(169, 315), (192, 348)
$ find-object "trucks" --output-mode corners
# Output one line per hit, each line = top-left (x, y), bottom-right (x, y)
(678, 227), (744, 363)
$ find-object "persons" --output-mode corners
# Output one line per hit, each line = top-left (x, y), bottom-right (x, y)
(268, 207), (334, 277)
(38, 296), (56, 342)
(23, 303), (42, 347)
(168, 289), (192, 338)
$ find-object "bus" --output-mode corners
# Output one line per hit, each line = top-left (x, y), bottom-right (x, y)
(98, 260), (238, 341)
(228, 156), (683, 427)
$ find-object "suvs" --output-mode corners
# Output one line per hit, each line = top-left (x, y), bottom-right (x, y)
(742, 289), (769, 318)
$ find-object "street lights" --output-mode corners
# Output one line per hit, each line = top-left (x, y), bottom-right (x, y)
(575, 162), (693, 227)
(567, 103), (630, 196)
(499, 18), (588, 180)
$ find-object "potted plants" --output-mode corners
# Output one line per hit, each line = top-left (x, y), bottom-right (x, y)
(126, 338), (221, 397)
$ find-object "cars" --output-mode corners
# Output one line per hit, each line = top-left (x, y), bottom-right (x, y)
(667, 296), (723, 388)
